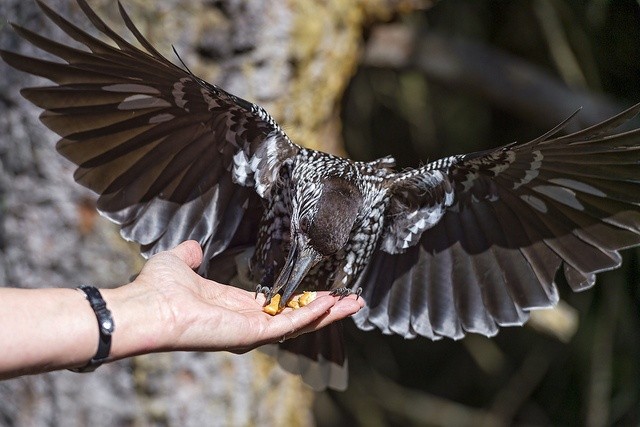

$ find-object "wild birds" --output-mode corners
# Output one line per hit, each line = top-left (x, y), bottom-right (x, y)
(0, 2), (640, 393)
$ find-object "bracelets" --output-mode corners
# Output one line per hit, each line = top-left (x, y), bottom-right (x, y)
(65, 279), (115, 373)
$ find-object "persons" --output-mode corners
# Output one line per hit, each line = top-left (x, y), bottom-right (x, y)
(1, 239), (366, 379)
